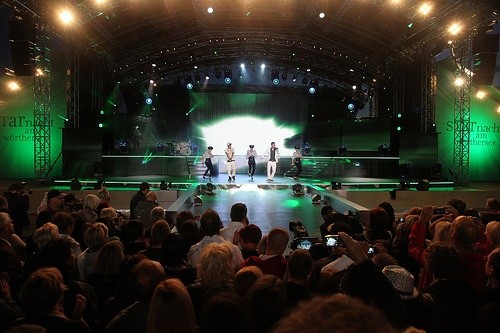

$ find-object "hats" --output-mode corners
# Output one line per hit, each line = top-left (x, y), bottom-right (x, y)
(447, 199), (466, 214)
(382, 265), (418, 300)
(208, 146), (213, 149)
(227, 142), (232, 146)
(294, 146), (300, 149)
(47, 189), (67, 200)
(249, 145), (254, 147)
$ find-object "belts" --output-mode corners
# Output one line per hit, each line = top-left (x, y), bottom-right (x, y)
(269, 160), (276, 161)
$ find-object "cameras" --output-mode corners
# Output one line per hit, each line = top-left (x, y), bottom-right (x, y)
(113, 217), (120, 223)
(324, 235), (345, 247)
(433, 207), (445, 215)
(367, 245), (377, 254)
(399, 217), (403, 222)
(289, 222), (302, 232)
(20, 182), (32, 196)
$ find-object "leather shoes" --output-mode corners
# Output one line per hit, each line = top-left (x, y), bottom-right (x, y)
(228, 160), (235, 162)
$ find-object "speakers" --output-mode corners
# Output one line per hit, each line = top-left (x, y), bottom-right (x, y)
(417, 179), (429, 191)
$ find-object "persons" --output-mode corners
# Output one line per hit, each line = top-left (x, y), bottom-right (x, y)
(292, 146), (303, 179)
(261, 142), (279, 182)
(246, 144), (257, 180)
(0, 182), (500, 333)
(225, 142), (237, 181)
(202, 146), (213, 180)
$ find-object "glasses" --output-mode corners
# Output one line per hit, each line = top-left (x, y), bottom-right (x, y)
(484, 256), (492, 265)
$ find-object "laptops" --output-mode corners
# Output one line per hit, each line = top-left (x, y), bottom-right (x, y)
(295, 237), (317, 250)
